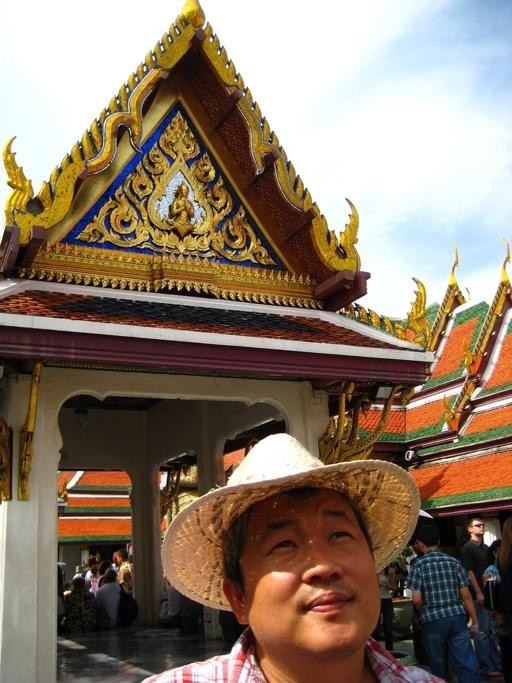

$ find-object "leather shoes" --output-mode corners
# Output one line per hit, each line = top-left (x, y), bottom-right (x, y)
(488, 669), (501, 676)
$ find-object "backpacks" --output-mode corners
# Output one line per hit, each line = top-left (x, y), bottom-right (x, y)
(117, 583), (138, 626)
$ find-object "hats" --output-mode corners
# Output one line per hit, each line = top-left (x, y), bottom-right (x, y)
(70, 576), (91, 591)
(160, 431), (421, 612)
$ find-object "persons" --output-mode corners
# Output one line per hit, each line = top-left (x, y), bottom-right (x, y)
(375, 514), (512, 683)
(59, 545), (197, 631)
(170, 181), (195, 226)
(141, 433), (446, 683)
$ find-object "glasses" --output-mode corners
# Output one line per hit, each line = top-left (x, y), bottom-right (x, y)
(474, 523), (485, 526)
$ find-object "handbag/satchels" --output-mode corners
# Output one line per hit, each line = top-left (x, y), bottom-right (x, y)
(482, 552), (501, 610)
(96, 606), (110, 629)
